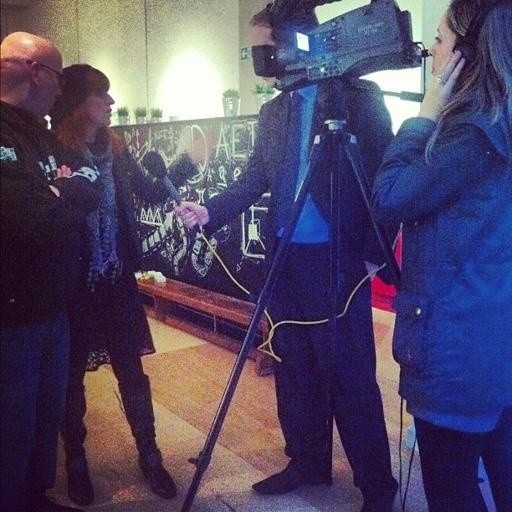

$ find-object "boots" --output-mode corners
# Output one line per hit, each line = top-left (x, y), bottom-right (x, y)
(58, 385), (98, 507)
(116, 375), (180, 500)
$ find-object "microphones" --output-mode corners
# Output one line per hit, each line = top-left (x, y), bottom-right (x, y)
(139, 148), (202, 235)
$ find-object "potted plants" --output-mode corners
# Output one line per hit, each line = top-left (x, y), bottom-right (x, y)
(115, 105), (164, 126)
(248, 83), (275, 113)
(223, 84), (241, 118)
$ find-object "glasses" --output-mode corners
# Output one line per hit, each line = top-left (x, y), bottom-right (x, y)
(35, 61), (69, 90)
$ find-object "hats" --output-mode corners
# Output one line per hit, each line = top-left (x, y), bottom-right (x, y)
(46, 61), (110, 117)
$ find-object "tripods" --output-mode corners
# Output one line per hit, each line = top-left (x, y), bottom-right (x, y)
(181, 121), (404, 512)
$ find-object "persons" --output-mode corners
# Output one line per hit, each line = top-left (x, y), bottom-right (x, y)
(368, 0), (512, 512)
(175, 2), (400, 512)
(49, 62), (220, 505)
(0, 31), (105, 512)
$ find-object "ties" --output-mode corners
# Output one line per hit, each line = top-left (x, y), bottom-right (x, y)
(275, 90), (304, 230)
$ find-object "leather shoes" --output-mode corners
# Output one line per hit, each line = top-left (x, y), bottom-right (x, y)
(362, 477), (399, 511)
(250, 456), (334, 494)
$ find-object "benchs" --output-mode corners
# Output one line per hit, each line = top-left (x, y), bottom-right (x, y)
(137, 277), (269, 361)
